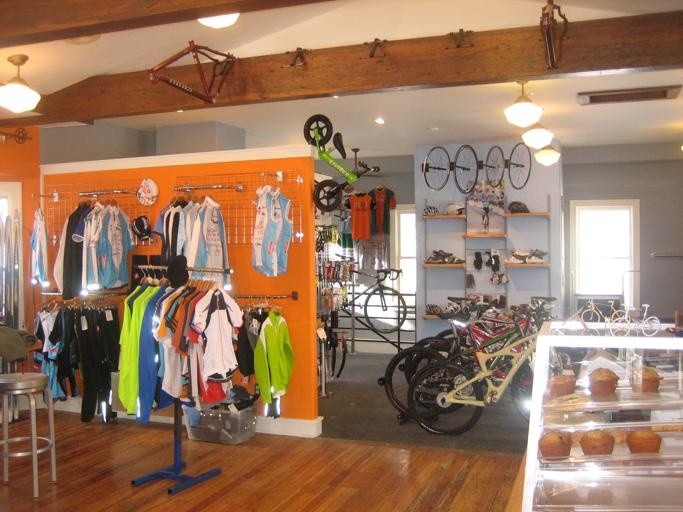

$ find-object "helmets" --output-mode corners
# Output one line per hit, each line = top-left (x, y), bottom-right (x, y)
(443, 203), (466, 215)
(423, 207), (439, 216)
(508, 201), (530, 213)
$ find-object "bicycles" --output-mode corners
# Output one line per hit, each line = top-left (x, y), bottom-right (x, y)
(334, 252), (406, 334)
(302, 114), (379, 212)
(377, 296), (563, 436)
(580, 296), (659, 336)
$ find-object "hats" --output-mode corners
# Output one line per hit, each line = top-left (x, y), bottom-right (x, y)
(167, 256), (189, 288)
(136, 178), (160, 206)
(130, 215), (151, 240)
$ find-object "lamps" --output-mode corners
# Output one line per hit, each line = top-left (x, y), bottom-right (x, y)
(502, 80), (562, 167)
(0, 54), (42, 114)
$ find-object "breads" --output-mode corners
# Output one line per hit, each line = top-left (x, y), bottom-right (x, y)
(580, 430), (614, 454)
(590, 374), (616, 394)
(549, 374), (577, 394)
(587, 368), (618, 384)
(626, 429), (661, 454)
(539, 428), (571, 461)
(628, 368), (659, 392)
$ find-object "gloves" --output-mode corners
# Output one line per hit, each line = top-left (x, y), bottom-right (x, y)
(465, 275), (475, 288)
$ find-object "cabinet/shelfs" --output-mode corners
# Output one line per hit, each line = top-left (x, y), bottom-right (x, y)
(521, 324), (683, 512)
(421, 210), (551, 322)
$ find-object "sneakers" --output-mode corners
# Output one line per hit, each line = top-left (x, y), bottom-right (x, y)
(510, 249), (546, 263)
(423, 249), (465, 264)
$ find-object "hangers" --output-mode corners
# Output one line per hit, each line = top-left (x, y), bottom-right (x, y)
(125, 264), (235, 306)
(159, 185), (224, 217)
(65, 191), (132, 221)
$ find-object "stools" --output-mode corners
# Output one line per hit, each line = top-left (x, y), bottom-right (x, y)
(0, 372), (57, 502)
